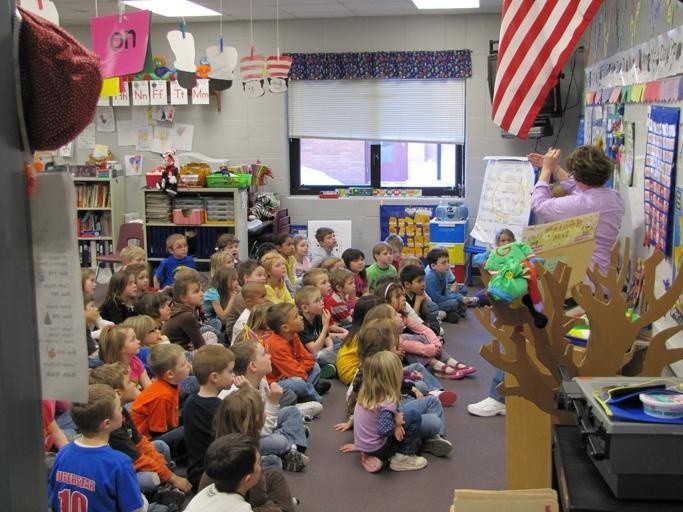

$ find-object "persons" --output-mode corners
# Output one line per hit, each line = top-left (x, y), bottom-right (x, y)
(335, 295), (385, 384)
(345, 304), (457, 407)
(424, 247), (468, 323)
(295, 285), (338, 378)
(210, 251), (234, 278)
(98, 324), (152, 396)
(400, 255), (432, 303)
(353, 351), (427, 473)
(89, 361), (192, 505)
(225, 261), (266, 338)
(425, 245), (480, 308)
(261, 302), (331, 404)
(384, 234), (404, 271)
(230, 282), (267, 346)
(323, 269), (356, 326)
(274, 233), (296, 286)
(42, 399), (69, 474)
(182, 345), (283, 494)
(84, 293), (99, 367)
(120, 245), (146, 269)
(293, 235), (312, 286)
(161, 271), (218, 350)
(217, 233), (243, 269)
(124, 263), (149, 292)
(365, 243), (397, 292)
(81, 267), (114, 340)
(342, 248), (369, 296)
(468, 145), (624, 414)
(182, 433), (262, 512)
(198, 387), (300, 512)
(399, 264), (445, 346)
(333, 320), (453, 458)
(308, 227), (337, 268)
(476, 228), (515, 305)
(101, 271), (140, 323)
(128, 344), (191, 457)
(47, 383), (149, 512)
(262, 252), (295, 305)
(258, 242), (295, 298)
(241, 302), (275, 342)
(320, 257), (344, 273)
(123, 315), (169, 347)
(154, 234), (195, 289)
(202, 268), (242, 330)
(373, 281), (477, 380)
(139, 293), (170, 326)
(218, 340), (310, 473)
(301, 269), (349, 338)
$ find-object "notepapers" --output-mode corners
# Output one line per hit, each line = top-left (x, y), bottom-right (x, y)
(237, 159), (274, 186)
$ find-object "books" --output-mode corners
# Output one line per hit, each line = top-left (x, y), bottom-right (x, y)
(75, 184), (111, 267)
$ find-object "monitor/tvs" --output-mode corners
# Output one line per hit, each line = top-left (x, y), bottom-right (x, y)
(488, 54), (561, 112)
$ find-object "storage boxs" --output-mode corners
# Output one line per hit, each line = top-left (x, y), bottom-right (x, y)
(206, 174), (252, 188)
(145, 173), (162, 186)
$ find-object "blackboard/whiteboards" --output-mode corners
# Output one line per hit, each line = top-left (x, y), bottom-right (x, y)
(584, 90), (682, 379)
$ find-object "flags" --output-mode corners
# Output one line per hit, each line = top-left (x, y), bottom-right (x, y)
(488, 0), (602, 140)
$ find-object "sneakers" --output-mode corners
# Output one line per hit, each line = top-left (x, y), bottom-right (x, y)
(361, 454), (382, 473)
(429, 390), (457, 408)
(467, 397), (506, 417)
(422, 433), (452, 457)
(442, 313), (460, 322)
(464, 296), (479, 305)
(280, 447), (310, 472)
(320, 364), (336, 378)
(295, 401), (323, 424)
(440, 326), (444, 336)
(438, 311), (447, 319)
(315, 380), (332, 395)
(389, 452), (428, 472)
(150, 486), (185, 507)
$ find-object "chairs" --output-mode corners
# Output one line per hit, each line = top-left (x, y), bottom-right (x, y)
(94, 223), (144, 286)
(249, 208), (291, 255)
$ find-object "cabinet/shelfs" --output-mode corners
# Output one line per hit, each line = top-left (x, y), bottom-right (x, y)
(73, 175), (126, 271)
(142, 187), (247, 280)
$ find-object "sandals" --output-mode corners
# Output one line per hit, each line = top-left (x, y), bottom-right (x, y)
(434, 365), (464, 379)
(450, 361), (476, 375)
